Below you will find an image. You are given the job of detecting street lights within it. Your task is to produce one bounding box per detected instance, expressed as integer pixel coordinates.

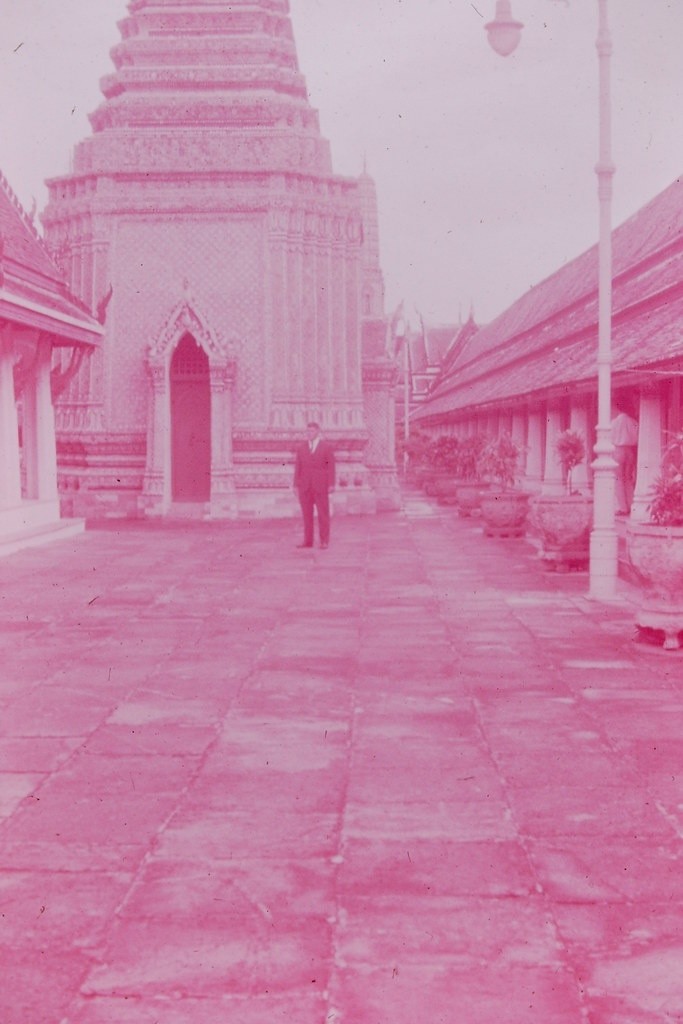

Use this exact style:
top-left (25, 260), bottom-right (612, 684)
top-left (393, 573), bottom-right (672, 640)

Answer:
top-left (485, 0), bottom-right (619, 599)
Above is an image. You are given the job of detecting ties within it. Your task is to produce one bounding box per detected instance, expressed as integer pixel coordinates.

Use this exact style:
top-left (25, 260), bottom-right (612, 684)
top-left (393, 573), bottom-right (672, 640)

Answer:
top-left (310, 440), bottom-right (314, 451)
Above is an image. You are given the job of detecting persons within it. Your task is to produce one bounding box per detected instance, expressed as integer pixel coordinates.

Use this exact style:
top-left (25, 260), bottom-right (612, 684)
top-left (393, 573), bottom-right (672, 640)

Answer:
top-left (294, 423), bottom-right (336, 549)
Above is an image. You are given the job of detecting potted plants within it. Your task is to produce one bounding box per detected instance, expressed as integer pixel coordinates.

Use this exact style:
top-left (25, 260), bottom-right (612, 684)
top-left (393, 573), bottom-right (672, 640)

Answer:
top-left (402, 425), bottom-right (682, 652)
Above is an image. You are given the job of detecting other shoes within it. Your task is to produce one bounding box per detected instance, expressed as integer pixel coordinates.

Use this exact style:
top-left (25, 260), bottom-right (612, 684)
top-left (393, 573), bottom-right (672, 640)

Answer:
top-left (296, 542), bottom-right (313, 548)
top-left (614, 509), bottom-right (631, 515)
top-left (320, 543), bottom-right (329, 549)
